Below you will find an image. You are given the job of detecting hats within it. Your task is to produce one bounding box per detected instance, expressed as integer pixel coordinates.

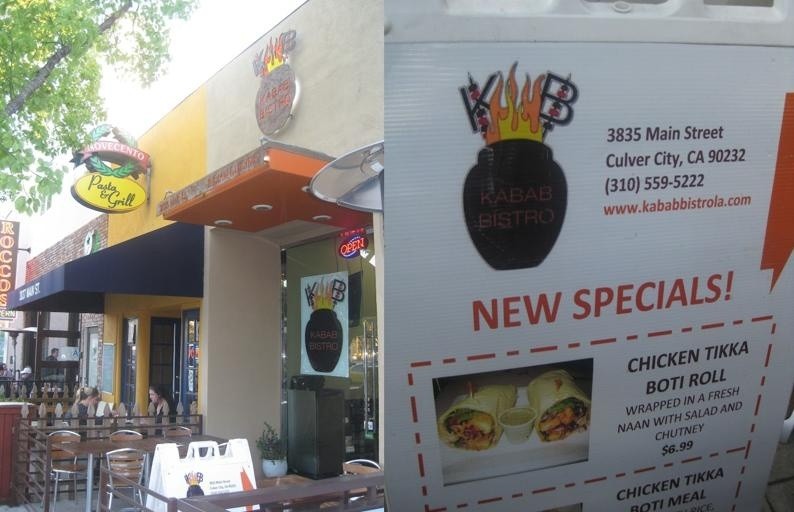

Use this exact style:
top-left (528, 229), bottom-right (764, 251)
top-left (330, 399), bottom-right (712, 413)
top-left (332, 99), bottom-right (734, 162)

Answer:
top-left (18, 367), bottom-right (32, 374)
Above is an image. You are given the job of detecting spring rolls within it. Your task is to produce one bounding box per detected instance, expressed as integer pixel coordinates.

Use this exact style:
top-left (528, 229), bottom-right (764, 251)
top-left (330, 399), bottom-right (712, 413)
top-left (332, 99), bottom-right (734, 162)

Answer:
top-left (528, 369), bottom-right (591, 442)
top-left (437, 383), bottom-right (517, 451)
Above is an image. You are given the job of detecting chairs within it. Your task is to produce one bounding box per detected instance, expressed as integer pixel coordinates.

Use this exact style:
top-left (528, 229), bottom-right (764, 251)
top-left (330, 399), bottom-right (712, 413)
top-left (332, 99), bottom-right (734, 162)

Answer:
top-left (41, 423), bottom-right (380, 511)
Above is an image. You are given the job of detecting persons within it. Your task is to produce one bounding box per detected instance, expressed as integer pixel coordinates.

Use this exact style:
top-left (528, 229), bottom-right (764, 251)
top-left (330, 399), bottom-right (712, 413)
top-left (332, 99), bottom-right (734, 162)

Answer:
top-left (16, 365), bottom-right (35, 395)
top-left (0, 363), bottom-right (12, 378)
top-left (61, 385), bottom-right (117, 487)
top-left (44, 348), bottom-right (59, 361)
top-left (140, 383), bottom-right (175, 435)
